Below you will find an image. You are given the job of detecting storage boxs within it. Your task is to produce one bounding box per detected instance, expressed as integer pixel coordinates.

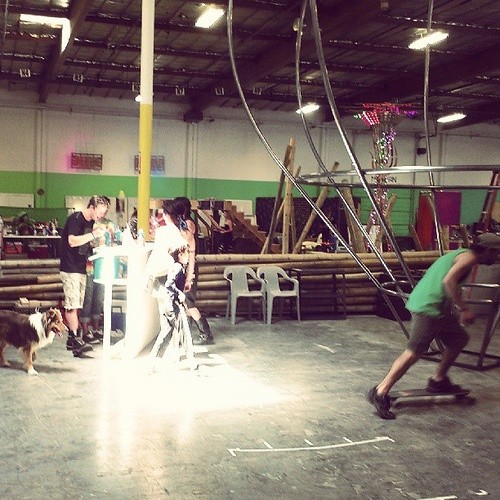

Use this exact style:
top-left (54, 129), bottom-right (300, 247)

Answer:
top-left (376, 274), bottom-right (423, 321)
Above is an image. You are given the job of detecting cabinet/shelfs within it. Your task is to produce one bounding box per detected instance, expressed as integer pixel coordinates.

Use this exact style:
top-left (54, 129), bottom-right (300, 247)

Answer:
top-left (288, 267), bottom-right (346, 320)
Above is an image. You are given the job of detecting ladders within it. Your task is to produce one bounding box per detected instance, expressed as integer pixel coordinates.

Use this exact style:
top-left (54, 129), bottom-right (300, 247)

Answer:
top-left (477, 170), bottom-right (500, 232)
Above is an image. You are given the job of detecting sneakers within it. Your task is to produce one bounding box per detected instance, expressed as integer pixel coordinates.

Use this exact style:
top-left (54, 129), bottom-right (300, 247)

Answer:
top-left (428, 375), bottom-right (460, 393)
top-left (368, 385), bottom-right (395, 419)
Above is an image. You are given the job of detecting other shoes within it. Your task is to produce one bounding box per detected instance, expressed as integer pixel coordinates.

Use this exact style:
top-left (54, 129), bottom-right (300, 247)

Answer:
top-left (83, 332), bottom-right (99, 344)
top-left (72, 337), bottom-right (93, 357)
top-left (67, 336), bottom-right (74, 348)
top-left (94, 329), bottom-right (104, 340)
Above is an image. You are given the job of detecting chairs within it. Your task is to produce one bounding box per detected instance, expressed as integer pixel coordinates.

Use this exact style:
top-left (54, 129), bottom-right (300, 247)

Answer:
top-left (461, 224), bottom-right (473, 248)
top-left (223, 266), bottom-right (266, 326)
top-left (442, 225), bottom-right (464, 250)
top-left (256, 266), bottom-right (300, 325)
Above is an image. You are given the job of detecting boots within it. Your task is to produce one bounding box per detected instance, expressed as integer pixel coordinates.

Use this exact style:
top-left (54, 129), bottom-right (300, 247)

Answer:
top-left (193, 315), bottom-right (213, 344)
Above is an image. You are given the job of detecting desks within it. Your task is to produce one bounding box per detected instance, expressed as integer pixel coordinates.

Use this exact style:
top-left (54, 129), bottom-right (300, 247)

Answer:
top-left (3, 235), bottom-right (62, 259)
top-left (302, 241), bottom-right (321, 250)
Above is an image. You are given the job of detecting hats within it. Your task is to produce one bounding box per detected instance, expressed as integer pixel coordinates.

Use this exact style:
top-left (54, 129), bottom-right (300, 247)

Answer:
top-left (473, 232), bottom-right (500, 249)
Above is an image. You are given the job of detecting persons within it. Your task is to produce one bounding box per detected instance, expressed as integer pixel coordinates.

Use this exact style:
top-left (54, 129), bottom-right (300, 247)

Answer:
top-left (366, 233), bottom-right (500, 420)
top-left (147, 197), bottom-right (200, 373)
top-left (172, 197), bottom-right (214, 344)
top-left (216, 211), bottom-right (234, 254)
top-left (57, 193), bottom-right (112, 357)
top-left (79, 225), bottom-right (107, 344)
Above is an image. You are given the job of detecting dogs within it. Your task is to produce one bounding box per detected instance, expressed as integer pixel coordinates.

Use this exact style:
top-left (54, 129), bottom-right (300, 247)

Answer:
top-left (0, 306), bottom-right (69, 376)
